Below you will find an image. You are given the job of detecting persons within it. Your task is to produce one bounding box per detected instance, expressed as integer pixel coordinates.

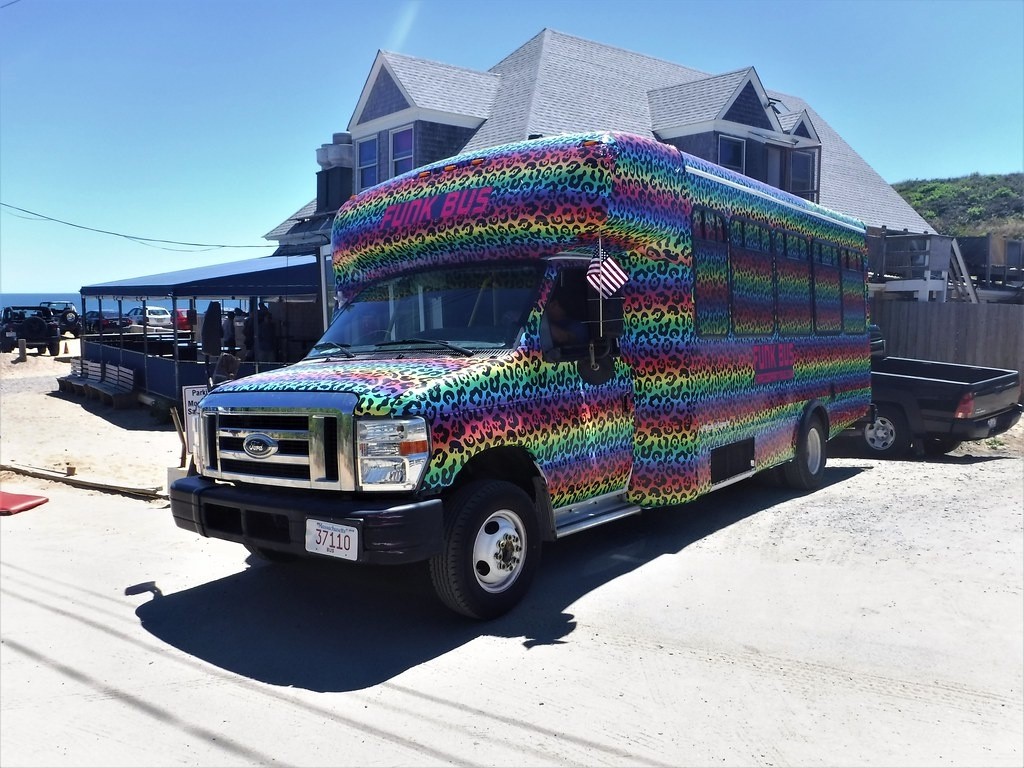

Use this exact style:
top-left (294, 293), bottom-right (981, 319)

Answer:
top-left (499, 270), bottom-right (608, 360)
top-left (243, 312), bottom-right (255, 365)
top-left (222, 311), bottom-right (237, 357)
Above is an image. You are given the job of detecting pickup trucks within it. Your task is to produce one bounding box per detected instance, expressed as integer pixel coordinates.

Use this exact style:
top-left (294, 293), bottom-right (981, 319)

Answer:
top-left (849, 325), bottom-right (1024, 459)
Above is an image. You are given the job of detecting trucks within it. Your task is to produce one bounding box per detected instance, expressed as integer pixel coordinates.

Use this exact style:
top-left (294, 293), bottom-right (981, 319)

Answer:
top-left (164, 125), bottom-right (875, 626)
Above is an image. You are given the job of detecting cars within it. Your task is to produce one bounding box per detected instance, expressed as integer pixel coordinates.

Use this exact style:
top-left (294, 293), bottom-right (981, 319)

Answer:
top-left (168, 309), bottom-right (190, 330)
top-left (79, 309), bottom-right (133, 334)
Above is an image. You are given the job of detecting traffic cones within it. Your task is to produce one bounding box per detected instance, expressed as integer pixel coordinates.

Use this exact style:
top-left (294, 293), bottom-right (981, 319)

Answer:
top-left (63, 341), bottom-right (69, 354)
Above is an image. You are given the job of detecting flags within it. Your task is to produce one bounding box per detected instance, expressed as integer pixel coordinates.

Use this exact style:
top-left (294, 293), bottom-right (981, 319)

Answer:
top-left (585, 240), bottom-right (630, 300)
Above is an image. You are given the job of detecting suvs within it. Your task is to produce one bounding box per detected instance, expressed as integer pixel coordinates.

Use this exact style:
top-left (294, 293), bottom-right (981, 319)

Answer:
top-left (34, 301), bottom-right (83, 338)
top-left (124, 306), bottom-right (173, 329)
top-left (0, 305), bottom-right (61, 356)
